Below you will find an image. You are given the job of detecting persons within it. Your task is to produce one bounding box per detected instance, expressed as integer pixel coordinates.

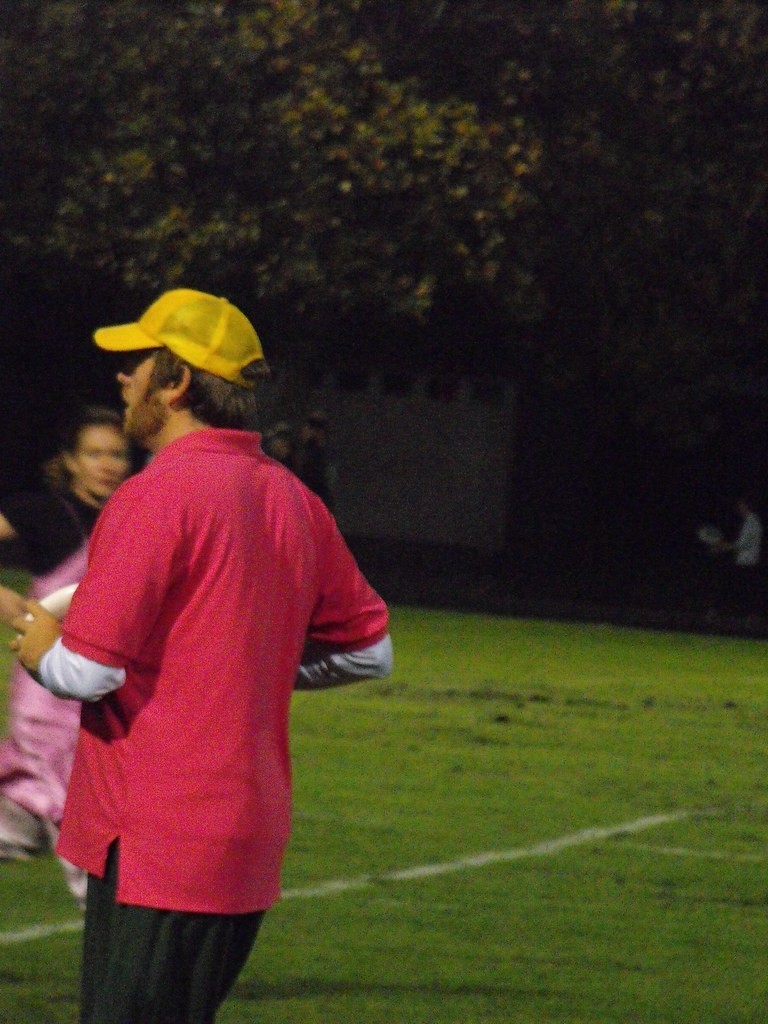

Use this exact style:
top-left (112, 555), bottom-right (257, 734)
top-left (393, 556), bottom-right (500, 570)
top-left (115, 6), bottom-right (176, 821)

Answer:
top-left (0, 289), bottom-right (392, 1024)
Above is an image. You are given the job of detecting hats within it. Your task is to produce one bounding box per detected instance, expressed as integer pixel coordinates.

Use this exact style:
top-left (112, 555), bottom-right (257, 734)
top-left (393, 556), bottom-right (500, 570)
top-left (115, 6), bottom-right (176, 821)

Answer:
top-left (94, 288), bottom-right (264, 391)
